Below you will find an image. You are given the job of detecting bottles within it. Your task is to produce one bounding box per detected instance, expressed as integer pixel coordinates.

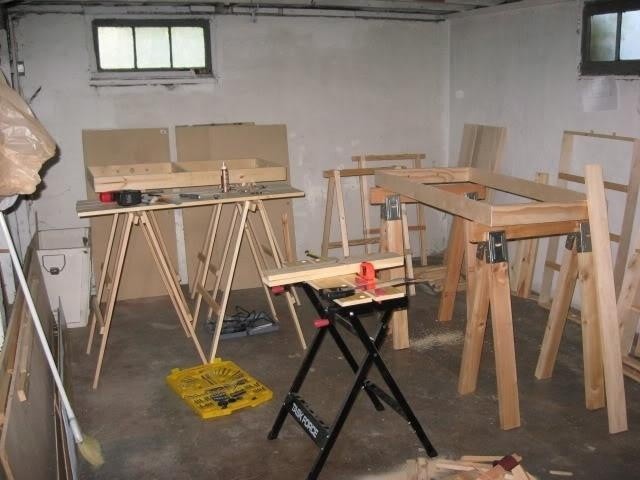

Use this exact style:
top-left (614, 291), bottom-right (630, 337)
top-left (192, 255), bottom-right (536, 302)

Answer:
top-left (220, 162), bottom-right (229, 193)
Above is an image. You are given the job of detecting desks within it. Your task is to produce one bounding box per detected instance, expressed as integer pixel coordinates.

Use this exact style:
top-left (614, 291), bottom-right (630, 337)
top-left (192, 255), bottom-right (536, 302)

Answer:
top-left (76, 181), bottom-right (307, 389)
top-left (269, 256), bottom-right (438, 479)
